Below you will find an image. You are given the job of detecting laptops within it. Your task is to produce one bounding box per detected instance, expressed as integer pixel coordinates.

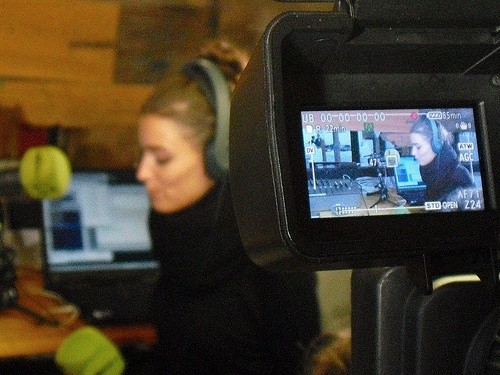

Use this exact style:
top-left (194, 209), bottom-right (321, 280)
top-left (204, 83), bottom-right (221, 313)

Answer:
top-left (393, 155), bottom-right (434, 207)
top-left (39, 171), bottom-right (166, 328)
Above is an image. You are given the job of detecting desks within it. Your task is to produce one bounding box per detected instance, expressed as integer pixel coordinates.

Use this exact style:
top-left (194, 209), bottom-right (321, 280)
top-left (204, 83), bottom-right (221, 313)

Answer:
top-left (0, 268), bottom-right (160, 359)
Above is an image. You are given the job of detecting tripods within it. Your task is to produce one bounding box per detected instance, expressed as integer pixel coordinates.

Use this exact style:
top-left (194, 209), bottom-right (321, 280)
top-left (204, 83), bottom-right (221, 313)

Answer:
top-left (367, 173), bottom-right (401, 209)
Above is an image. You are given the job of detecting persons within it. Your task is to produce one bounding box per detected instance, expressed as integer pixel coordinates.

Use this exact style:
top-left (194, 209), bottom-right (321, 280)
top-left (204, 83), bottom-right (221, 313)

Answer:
top-left (409, 114), bottom-right (476, 206)
top-left (119, 40), bottom-right (322, 375)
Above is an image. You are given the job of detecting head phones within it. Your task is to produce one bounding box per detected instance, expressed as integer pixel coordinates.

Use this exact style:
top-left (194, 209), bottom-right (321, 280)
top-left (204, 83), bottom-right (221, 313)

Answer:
top-left (423, 116), bottom-right (445, 152)
top-left (187, 59), bottom-right (234, 179)
